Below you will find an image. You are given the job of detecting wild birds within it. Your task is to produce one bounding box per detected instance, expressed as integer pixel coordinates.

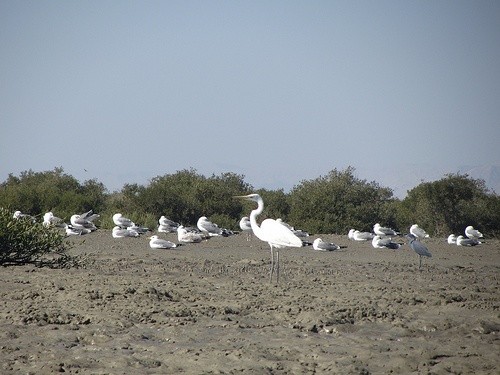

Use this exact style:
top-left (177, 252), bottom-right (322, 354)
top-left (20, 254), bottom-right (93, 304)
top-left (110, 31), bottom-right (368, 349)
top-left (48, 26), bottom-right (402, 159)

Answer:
top-left (233, 194), bottom-right (303, 286)
top-left (12, 209), bottom-right (483, 269)
